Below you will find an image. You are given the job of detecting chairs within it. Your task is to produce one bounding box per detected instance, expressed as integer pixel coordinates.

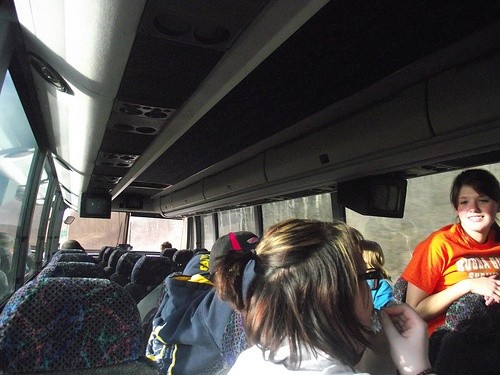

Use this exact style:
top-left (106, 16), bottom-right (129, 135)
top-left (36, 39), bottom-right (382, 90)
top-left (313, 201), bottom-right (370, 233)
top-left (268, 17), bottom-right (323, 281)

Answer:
top-left (0, 247), bottom-right (500, 375)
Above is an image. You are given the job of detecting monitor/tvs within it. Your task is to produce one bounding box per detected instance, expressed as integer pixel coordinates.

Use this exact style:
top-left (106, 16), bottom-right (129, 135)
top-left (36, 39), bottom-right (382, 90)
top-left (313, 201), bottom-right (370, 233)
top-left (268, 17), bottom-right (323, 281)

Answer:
top-left (80, 191), bottom-right (111, 219)
top-left (336, 173), bottom-right (407, 218)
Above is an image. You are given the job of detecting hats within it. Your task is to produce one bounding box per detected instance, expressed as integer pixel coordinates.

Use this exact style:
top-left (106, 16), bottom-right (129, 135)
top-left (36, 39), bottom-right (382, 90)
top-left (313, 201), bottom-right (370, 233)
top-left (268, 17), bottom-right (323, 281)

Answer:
top-left (210, 231), bottom-right (261, 274)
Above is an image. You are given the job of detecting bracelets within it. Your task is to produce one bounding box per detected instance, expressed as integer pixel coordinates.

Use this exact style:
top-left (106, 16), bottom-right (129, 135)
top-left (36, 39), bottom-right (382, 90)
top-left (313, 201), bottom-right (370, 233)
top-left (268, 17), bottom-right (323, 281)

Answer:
top-left (418, 368), bottom-right (437, 375)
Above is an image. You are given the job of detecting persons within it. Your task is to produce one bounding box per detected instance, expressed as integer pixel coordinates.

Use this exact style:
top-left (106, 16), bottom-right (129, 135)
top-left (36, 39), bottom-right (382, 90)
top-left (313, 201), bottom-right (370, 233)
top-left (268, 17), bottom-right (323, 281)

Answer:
top-left (161, 241), bottom-right (172, 252)
top-left (401, 168), bottom-right (500, 336)
top-left (0, 232), bottom-right (29, 275)
top-left (146, 231), bottom-right (261, 375)
top-left (360, 240), bottom-right (395, 314)
top-left (216, 218), bottom-right (437, 375)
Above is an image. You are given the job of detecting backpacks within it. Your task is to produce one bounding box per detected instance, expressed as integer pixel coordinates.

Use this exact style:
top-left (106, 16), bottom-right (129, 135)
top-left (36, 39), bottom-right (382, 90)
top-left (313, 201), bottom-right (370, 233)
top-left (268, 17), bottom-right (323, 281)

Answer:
top-left (152, 255), bottom-right (214, 328)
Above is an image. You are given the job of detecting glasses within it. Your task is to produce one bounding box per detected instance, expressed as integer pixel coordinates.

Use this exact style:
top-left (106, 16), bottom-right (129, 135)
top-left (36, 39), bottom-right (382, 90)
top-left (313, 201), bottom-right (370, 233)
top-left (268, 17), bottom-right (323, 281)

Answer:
top-left (357, 270), bottom-right (380, 291)
